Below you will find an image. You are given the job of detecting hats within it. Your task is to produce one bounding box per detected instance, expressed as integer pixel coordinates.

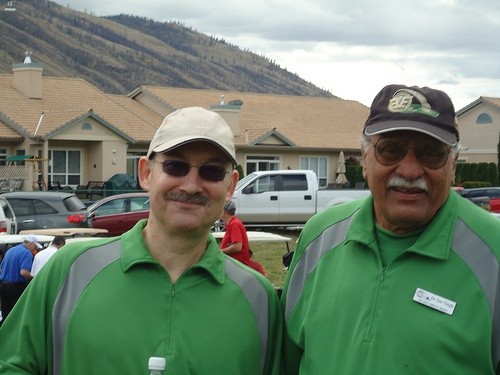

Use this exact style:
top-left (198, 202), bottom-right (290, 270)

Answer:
top-left (25, 235), bottom-right (43, 248)
top-left (147, 106), bottom-right (238, 168)
top-left (362, 84), bottom-right (460, 147)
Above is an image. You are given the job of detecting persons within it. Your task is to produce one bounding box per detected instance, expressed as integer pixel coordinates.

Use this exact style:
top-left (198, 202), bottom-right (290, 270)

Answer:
top-left (280, 84), bottom-right (500, 375)
top-left (0, 234), bottom-right (65, 326)
top-left (0, 106), bottom-right (279, 375)
top-left (220, 201), bottom-right (265, 275)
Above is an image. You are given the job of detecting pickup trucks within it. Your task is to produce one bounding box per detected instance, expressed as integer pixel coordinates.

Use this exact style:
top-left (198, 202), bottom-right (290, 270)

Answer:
top-left (222, 170), bottom-right (372, 230)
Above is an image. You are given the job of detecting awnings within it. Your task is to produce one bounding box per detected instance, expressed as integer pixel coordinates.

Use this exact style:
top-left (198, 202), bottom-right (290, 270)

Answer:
top-left (4, 155), bottom-right (34, 160)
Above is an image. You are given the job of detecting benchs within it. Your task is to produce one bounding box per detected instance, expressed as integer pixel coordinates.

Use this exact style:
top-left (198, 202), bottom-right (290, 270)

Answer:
top-left (38, 180), bottom-right (73, 193)
top-left (75, 181), bottom-right (106, 201)
top-left (0, 179), bottom-right (24, 194)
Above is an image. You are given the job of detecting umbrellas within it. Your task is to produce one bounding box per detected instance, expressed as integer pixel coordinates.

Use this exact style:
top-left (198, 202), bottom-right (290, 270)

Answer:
top-left (335, 150), bottom-right (349, 187)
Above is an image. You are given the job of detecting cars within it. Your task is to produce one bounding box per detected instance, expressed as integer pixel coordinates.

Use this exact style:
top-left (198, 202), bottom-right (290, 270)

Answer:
top-left (451, 186), bottom-right (500, 212)
top-left (87, 192), bottom-right (153, 237)
top-left (1, 192), bottom-right (89, 234)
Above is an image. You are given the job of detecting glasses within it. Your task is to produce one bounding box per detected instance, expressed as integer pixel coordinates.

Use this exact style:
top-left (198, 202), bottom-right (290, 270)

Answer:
top-left (153, 159), bottom-right (234, 182)
top-left (368, 137), bottom-right (452, 170)
